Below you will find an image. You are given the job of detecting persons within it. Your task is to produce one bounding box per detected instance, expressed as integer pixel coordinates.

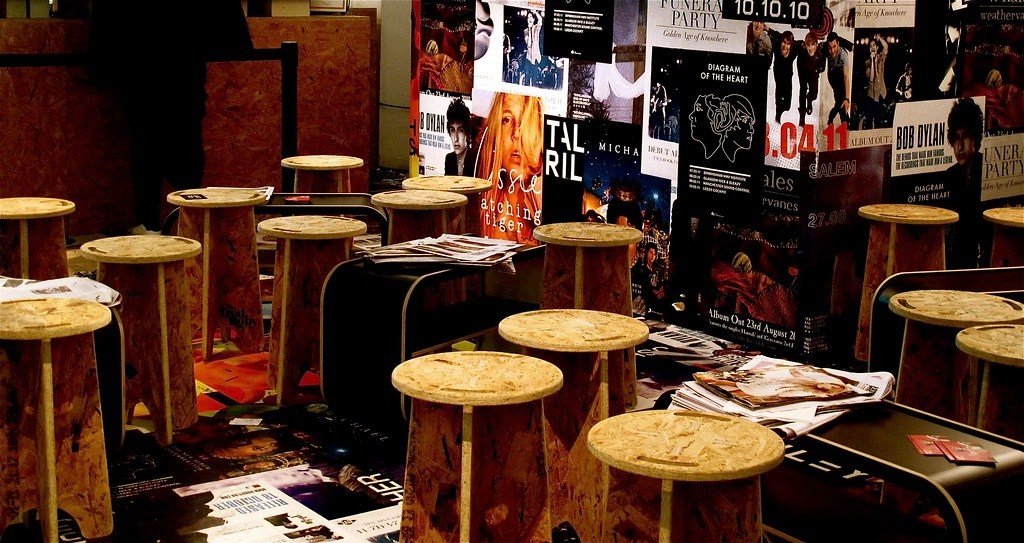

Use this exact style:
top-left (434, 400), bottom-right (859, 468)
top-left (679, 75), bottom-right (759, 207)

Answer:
top-left (944, 97), bottom-right (983, 201)
top-left (474, 92), bottom-right (544, 245)
top-left (524, 9), bottom-right (542, 86)
top-left (49, 0), bottom-right (257, 243)
top-left (746, 21), bottom-right (912, 130)
top-left (716, 365), bottom-right (849, 397)
top-left (634, 242), bottom-right (663, 321)
top-left (444, 97), bottom-right (483, 175)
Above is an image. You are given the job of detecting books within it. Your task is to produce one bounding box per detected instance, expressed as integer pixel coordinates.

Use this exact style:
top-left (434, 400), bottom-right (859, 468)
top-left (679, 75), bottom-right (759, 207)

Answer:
top-left (355, 233), bottom-right (526, 267)
top-left (693, 354), bottom-right (873, 410)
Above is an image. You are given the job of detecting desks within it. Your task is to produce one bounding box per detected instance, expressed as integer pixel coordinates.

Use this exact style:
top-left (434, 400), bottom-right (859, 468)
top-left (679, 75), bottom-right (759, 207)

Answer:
top-left (868, 267), bottom-right (1024, 401)
top-left (318, 234), bottom-right (546, 424)
top-left (0, 275), bottom-right (126, 450)
top-left (652, 388), bottom-right (1024, 543)
top-left (161, 193), bottom-right (389, 247)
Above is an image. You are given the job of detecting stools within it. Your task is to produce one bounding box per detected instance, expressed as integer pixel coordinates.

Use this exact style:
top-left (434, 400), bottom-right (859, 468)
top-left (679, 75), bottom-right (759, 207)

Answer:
top-left (854, 204), bottom-right (959, 362)
top-left (0, 197), bottom-right (75, 281)
top-left (889, 290), bottom-right (1024, 427)
top-left (402, 176), bottom-right (492, 235)
top-left (0, 298), bottom-right (113, 543)
top-left (956, 324), bottom-right (1024, 443)
top-left (983, 207), bottom-right (1024, 268)
top-left (586, 410), bottom-right (785, 543)
top-left (257, 215), bottom-right (367, 407)
top-left (392, 350), bottom-right (563, 543)
top-left (281, 155), bottom-right (364, 251)
top-left (371, 190), bottom-right (468, 246)
top-left (533, 222), bottom-right (644, 408)
top-left (167, 188), bottom-right (266, 360)
top-left (80, 235), bottom-right (203, 446)
top-left (499, 309), bottom-right (649, 543)
